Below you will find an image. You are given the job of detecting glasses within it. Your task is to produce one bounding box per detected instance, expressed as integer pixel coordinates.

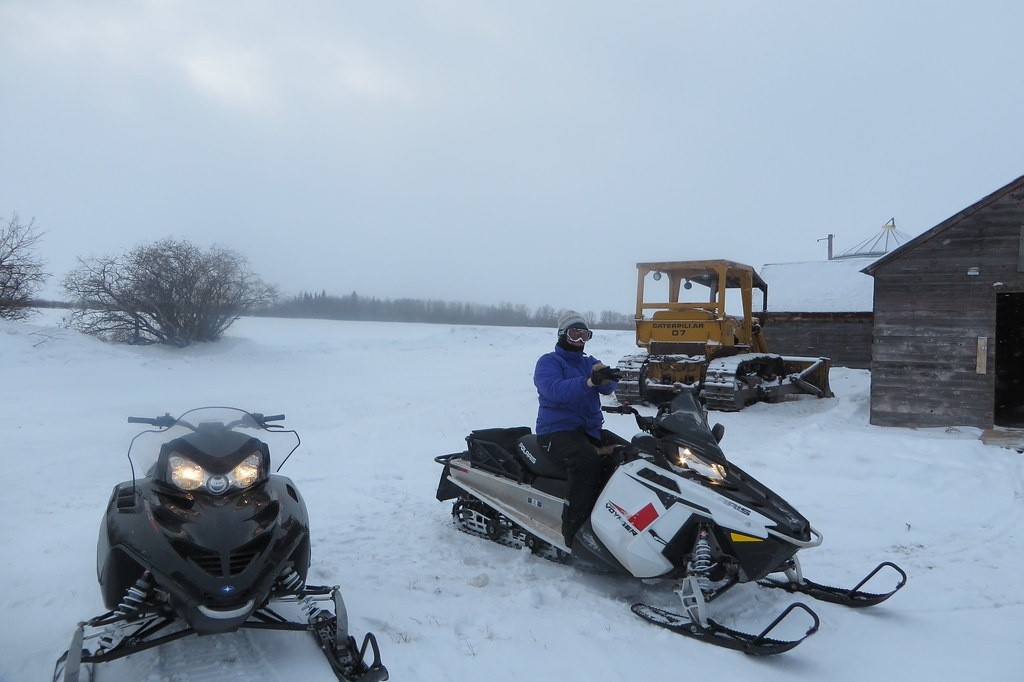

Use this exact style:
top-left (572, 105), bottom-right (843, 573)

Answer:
top-left (558, 327), bottom-right (593, 343)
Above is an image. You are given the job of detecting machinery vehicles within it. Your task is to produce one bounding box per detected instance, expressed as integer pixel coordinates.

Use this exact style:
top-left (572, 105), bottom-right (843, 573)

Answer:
top-left (612, 259), bottom-right (834, 413)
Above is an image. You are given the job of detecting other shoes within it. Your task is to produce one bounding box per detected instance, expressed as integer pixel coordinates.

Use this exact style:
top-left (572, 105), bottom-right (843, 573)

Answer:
top-left (565, 519), bottom-right (579, 533)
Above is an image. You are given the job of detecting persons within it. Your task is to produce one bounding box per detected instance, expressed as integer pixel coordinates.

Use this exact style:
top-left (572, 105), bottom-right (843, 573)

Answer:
top-left (533, 310), bottom-right (630, 548)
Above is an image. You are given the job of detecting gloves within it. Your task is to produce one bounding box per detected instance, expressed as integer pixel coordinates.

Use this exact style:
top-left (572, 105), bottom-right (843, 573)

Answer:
top-left (591, 366), bottom-right (622, 385)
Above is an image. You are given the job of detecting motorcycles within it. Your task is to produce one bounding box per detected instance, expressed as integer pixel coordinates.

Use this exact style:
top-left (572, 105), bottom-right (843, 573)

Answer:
top-left (434, 390), bottom-right (907, 656)
top-left (53, 408), bottom-right (389, 681)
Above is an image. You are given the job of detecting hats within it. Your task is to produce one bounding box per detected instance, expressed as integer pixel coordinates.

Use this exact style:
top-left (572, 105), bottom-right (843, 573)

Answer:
top-left (558, 310), bottom-right (588, 340)
